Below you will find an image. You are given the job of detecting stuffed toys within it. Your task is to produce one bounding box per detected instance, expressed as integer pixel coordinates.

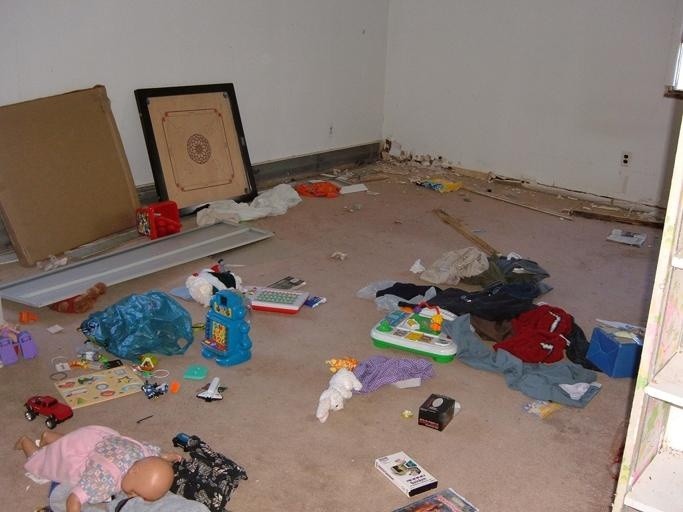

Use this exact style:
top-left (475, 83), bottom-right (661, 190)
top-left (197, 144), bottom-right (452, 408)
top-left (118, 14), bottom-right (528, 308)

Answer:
top-left (185, 259), bottom-right (243, 308)
top-left (46, 280), bottom-right (108, 314)
top-left (314, 366), bottom-right (362, 425)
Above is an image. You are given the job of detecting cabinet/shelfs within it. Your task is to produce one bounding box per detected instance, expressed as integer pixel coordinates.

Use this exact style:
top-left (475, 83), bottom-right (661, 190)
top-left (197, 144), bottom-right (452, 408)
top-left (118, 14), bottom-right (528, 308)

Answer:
top-left (622, 251), bottom-right (683, 512)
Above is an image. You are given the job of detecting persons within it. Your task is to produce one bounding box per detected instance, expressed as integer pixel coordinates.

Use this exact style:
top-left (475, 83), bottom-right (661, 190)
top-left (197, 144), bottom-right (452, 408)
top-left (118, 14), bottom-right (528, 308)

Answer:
top-left (12, 423), bottom-right (183, 511)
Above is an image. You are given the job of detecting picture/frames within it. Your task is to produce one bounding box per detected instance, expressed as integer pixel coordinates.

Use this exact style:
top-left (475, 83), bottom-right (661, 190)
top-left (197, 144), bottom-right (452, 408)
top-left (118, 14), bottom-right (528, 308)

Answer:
top-left (132, 82), bottom-right (257, 220)
